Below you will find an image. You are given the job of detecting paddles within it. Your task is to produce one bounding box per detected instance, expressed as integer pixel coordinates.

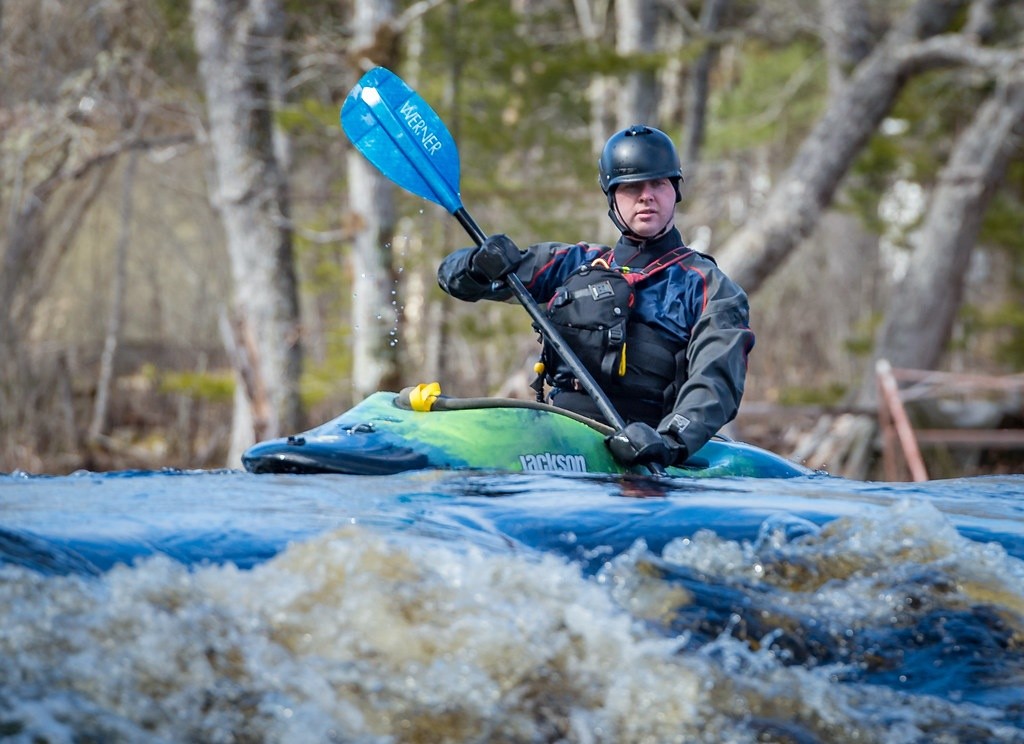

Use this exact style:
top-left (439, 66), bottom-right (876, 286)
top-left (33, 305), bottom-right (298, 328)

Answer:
top-left (335, 62), bottom-right (674, 482)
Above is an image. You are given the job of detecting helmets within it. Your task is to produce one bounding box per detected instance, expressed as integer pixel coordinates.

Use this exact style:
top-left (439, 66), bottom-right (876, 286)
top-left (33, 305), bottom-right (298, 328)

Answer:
top-left (598, 124), bottom-right (683, 204)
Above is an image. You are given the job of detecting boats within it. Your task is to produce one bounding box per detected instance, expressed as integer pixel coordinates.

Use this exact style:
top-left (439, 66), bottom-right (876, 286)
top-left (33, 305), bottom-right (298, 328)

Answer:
top-left (240, 388), bottom-right (816, 480)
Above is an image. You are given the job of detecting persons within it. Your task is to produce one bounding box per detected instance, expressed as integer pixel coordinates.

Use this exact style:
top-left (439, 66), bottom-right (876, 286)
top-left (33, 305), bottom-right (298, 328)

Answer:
top-left (439, 125), bottom-right (756, 468)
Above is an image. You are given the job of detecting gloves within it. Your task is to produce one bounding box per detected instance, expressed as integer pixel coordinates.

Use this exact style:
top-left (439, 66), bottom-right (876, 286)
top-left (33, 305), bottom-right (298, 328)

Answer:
top-left (466, 233), bottom-right (522, 292)
top-left (604, 422), bottom-right (687, 469)
